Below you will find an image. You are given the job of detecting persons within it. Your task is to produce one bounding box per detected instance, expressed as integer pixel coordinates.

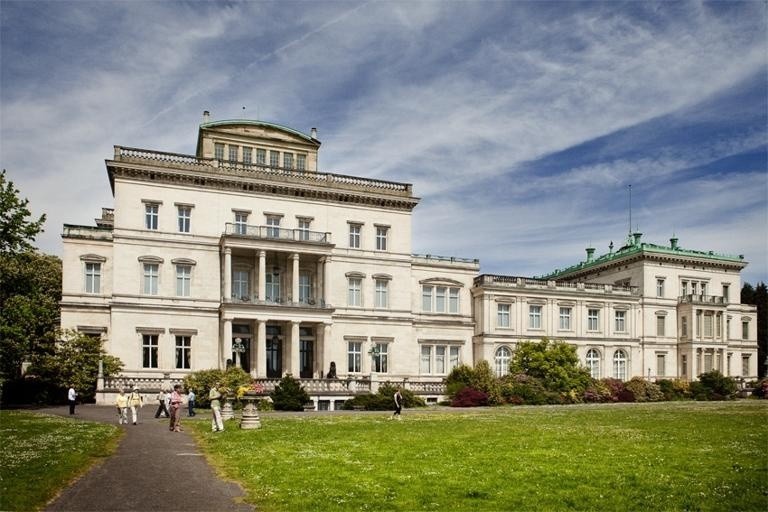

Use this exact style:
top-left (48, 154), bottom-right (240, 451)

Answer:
top-left (154, 388), bottom-right (171, 419)
top-left (392, 387), bottom-right (403, 416)
top-left (160, 389), bottom-right (172, 417)
top-left (127, 386), bottom-right (144, 426)
top-left (68, 382), bottom-right (77, 415)
top-left (168, 384), bottom-right (183, 431)
top-left (207, 381), bottom-right (227, 432)
top-left (186, 387), bottom-right (197, 416)
top-left (115, 387), bottom-right (129, 423)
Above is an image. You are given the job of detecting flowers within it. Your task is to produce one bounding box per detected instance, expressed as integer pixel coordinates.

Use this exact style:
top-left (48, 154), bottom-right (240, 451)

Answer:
top-left (218, 386), bottom-right (234, 394)
top-left (238, 383), bottom-right (264, 400)
